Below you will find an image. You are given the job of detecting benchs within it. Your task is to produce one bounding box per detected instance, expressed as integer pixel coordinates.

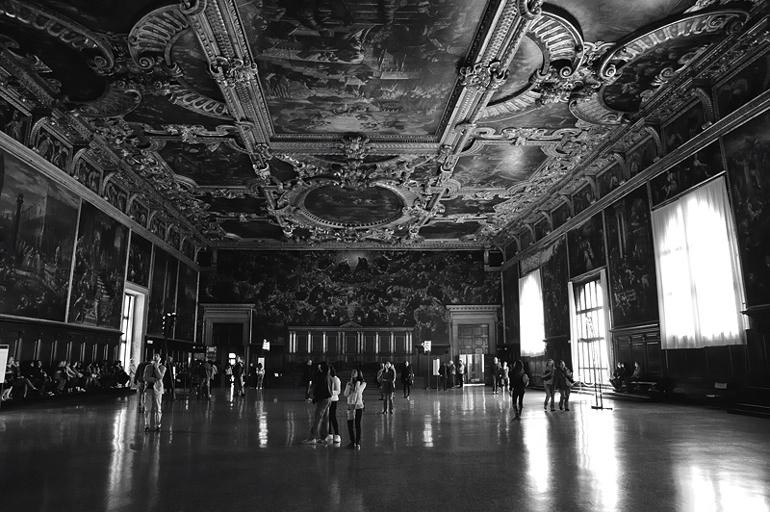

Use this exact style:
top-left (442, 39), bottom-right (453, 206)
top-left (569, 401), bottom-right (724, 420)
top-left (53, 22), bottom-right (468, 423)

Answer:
top-left (613, 378), bottom-right (657, 393)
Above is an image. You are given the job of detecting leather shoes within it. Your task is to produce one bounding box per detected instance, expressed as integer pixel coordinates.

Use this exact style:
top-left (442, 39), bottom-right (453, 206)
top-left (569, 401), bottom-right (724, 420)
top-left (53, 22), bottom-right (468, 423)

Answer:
top-left (144, 425), bottom-right (148, 431)
top-left (155, 425), bottom-right (160, 430)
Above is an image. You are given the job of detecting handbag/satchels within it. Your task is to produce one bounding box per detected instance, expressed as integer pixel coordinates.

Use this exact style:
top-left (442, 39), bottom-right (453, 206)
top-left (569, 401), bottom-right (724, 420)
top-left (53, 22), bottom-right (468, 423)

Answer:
top-left (523, 373), bottom-right (529, 385)
top-left (565, 376), bottom-right (573, 387)
top-left (346, 409), bottom-right (356, 421)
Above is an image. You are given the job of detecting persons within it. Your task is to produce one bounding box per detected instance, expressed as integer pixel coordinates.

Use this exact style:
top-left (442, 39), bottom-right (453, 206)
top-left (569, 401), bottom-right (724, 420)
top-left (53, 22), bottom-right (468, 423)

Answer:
top-left (344, 368), bottom-right (368, 451)
top-left (630, 360), bottom-right (641, 390)
top-left (0, 350), bottom-right (263, 436)
top-left (617, 363), bottom-right (627, 387)
top-left (324, 364), bottom-right (343, 444)
top-left (305, 359), bottom-right (574, 420)
top-left (305, 362), bottom-right (331, 445)
top-left (610, 361), bottom-right (620, 389)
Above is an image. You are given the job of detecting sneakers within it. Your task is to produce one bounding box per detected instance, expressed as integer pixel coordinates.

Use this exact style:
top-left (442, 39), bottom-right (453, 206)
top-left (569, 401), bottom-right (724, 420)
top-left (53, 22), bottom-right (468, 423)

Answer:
top-left (301, 431), bottom-right (361, 450)
